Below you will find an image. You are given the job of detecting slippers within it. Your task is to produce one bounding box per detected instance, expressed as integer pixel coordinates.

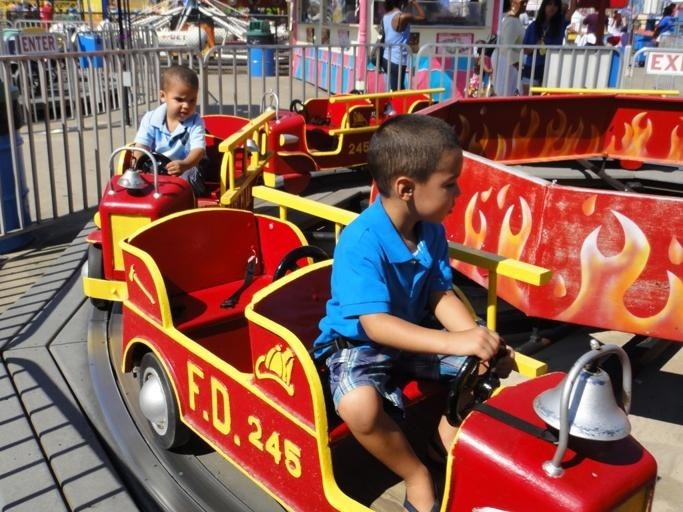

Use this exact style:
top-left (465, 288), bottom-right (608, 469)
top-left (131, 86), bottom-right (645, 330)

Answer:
top-left (402, 472), bottom-right (440, 512)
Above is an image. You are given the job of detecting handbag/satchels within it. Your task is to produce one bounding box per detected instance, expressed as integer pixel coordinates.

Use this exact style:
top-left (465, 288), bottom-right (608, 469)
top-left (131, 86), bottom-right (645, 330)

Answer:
top-left (371, 35), bottom-right (384, 67)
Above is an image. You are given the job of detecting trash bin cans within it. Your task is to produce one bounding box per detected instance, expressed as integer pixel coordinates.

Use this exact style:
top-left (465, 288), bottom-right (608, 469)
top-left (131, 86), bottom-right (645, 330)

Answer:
top-left (77, 33), bottom-right (104, 70)
top-left (243, 20), bottom-right (275, 77)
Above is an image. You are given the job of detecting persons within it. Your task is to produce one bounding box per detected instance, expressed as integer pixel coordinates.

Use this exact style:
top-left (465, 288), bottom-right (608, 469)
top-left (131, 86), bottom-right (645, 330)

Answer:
top-left (380, 0), bottom-right (427, 92)
top-left (132, 65), bottom-right (209, 194)
top-left (8, 0), bottom-right (83, 30)
top-left (312, 114), bottom-right (515, 512)
top-left (485, 0), bottom-right (683, 97)
